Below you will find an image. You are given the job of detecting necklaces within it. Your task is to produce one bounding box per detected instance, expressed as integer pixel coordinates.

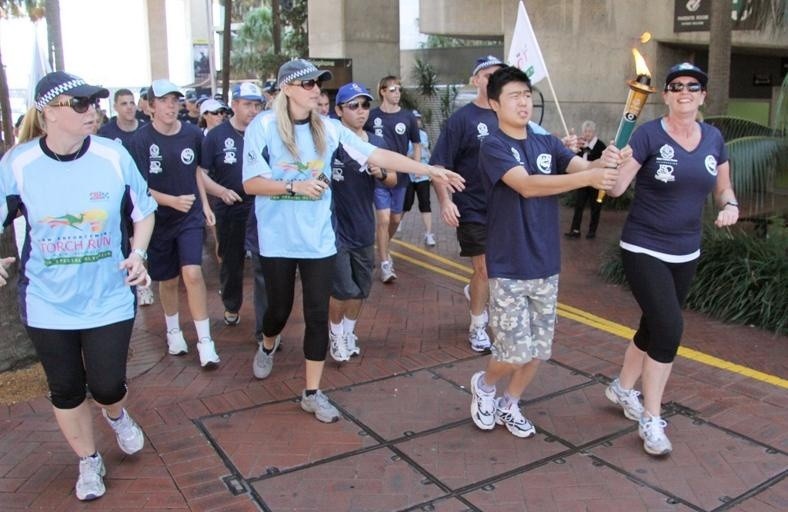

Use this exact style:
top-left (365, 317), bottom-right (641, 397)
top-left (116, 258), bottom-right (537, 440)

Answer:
top-left (46, 138), bottom-right (80, 174)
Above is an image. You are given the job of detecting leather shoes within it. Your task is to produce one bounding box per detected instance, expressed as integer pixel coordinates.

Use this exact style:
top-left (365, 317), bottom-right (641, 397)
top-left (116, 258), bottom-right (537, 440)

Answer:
top-left (586, 233), bottom-right (596, 239)
top-left (564, 230), bottom-right (581, 239)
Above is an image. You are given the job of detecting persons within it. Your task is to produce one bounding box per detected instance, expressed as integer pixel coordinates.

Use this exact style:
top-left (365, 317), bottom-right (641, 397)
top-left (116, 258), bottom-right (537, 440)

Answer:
top-left (199, 98), bottom-right (225, 265)
top-left (137, 87), bottom-right (153, 123)
top-left (329, 83), bottom-right (398, 363)
top-left (1, 72), bottom-right (158, 502)
top-left (363, 77), bottom-right (420, 283)
top-left (318, 89), bottom-right (339, 119)
top-left (564, 120), bottom-right (607, 241)
top-left (601, 62), bottom-right (738, 456)
top-left (200, 81), bottom-right (284, 351)
top-left (394, 109), bottom-right (437, 247)
top-left (428, 56), bottom-right (577, 353)
top-left (470, 66), bottom-right (634, 440)
top-left (96, 87), bottom-right (154, 307)
top-left (180, 90), bottom-right (199, 124)
top-left (130, 79), bottom-right (221, 371)
top-left (242, 57), bottom-right (465, 423)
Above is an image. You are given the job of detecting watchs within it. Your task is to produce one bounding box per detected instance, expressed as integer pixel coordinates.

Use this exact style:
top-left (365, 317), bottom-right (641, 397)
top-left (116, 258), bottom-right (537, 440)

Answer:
top-left (376, 167), bottom-right (388, 182)
top-left (132, 247), bottom-right (148, 260)
top-left (720, 201), bottom-right (738, 211)
top-left (285, 180), bottom-right (295, 197)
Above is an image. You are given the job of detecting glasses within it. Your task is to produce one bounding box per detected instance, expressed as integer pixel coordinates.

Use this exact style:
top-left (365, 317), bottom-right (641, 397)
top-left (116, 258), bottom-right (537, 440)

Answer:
top-left (664, 81), bottom-right (707, 92)
top-left (140, 96), bottom-right (149, 101)
top-left (207, 109), bottom-right (225, 116)
top-left (47, 96), bottom-right (98, 112)
top-left (380, 86), bottom-right (404, 94)
top-left (339, 101), bottom-right (373, 112)
top-left (287, 79), bottom-right (325, 93)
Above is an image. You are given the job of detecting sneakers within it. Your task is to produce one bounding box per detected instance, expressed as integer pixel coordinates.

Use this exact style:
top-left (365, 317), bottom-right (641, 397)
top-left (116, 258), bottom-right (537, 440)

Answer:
top-left (424, 233), bottom-right (436, 247)
top-left (468, 370), bottom-right (497, 432)
top-left (327, 330), bottom-right (352, 363)
top-left (463, 282), bottom-right (475, 312)
top-left (101, 404), bottom-right (146, 457)
top-left (467, 324), bottom-right (493, 354)
top-left (253, 333), bottom-right (282, 380)
top-left (344, 331), bottom-right (361, 358)
top-left (222, 310), bottom-right (241, 326)
top-left (638, 414), bottom-right (675, 458)
top-left (75, 449), bottom-right (107, 501)
top-left (165, 328), bottom-right (189, 357)
top-left (138, 288), bottom-right (154, 307)
top-left (380, 261), bottom-right (398, 284)
top-left (394, 219), bottom-right (405, 240)
top-left (605, 377), bottom-right (644, 423)
top-left (196, 340), bottom-right (222, 370)
top-left (301, 388), bottom-right (341, 425)
top-left (494, 396), bottom-right (538, 440)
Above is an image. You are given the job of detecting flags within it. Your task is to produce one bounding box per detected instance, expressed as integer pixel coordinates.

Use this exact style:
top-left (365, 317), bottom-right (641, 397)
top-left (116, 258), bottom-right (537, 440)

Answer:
top-left (501, 2), bottom-right (548, 87)
top-left (28, 35), bottom-right (52, 109)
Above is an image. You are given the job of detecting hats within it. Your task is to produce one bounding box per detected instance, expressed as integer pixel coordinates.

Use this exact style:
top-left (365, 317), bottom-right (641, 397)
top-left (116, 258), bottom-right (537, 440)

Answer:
top-left (199, 100), bottom-right (229, 117)
top-left (141, 87), bottom-right (153, 97)
top-left (472, 55), bottom-right (509, 75)
top-left (262, 81), bottom-right (279, 94)
top-left (145, 79), bottom-right (185, 107)
top-left (230, 81), bottom-right (265, 104)
top-left (663, 61), bottom-right (712, 87)
top-left (276, 57), bottom-right (335, 91)
top-left (335, 83), bottom-right (375, 107)
top-left (33, 71), bottom-right (109, 113)
